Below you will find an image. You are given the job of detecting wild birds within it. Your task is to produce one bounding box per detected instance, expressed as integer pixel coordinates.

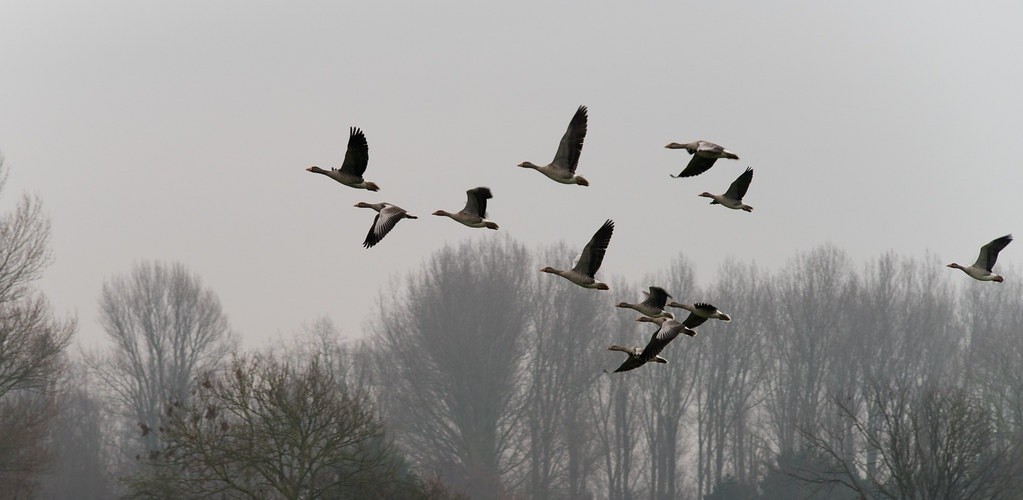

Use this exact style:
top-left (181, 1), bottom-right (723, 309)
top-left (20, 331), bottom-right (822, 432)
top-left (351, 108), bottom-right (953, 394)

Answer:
top-left (540, 221), bottom-right (616, 292)
top-left (945, 233), bottom-right (1014, 284)
top-left (352, 200), bottom-right (419, 250)
top-left (605, 285), bottom-right (732, 375)
top-left (516, 103), bottom-right (590, 187)
top-left (305, 125), bottom-right (381, 193)
top-left (431, 185), bottom-right (499, 231)
top-left (697, 166), bottom-right (754, 215)
top-left (663, 139), bottom-right (740, 178)
